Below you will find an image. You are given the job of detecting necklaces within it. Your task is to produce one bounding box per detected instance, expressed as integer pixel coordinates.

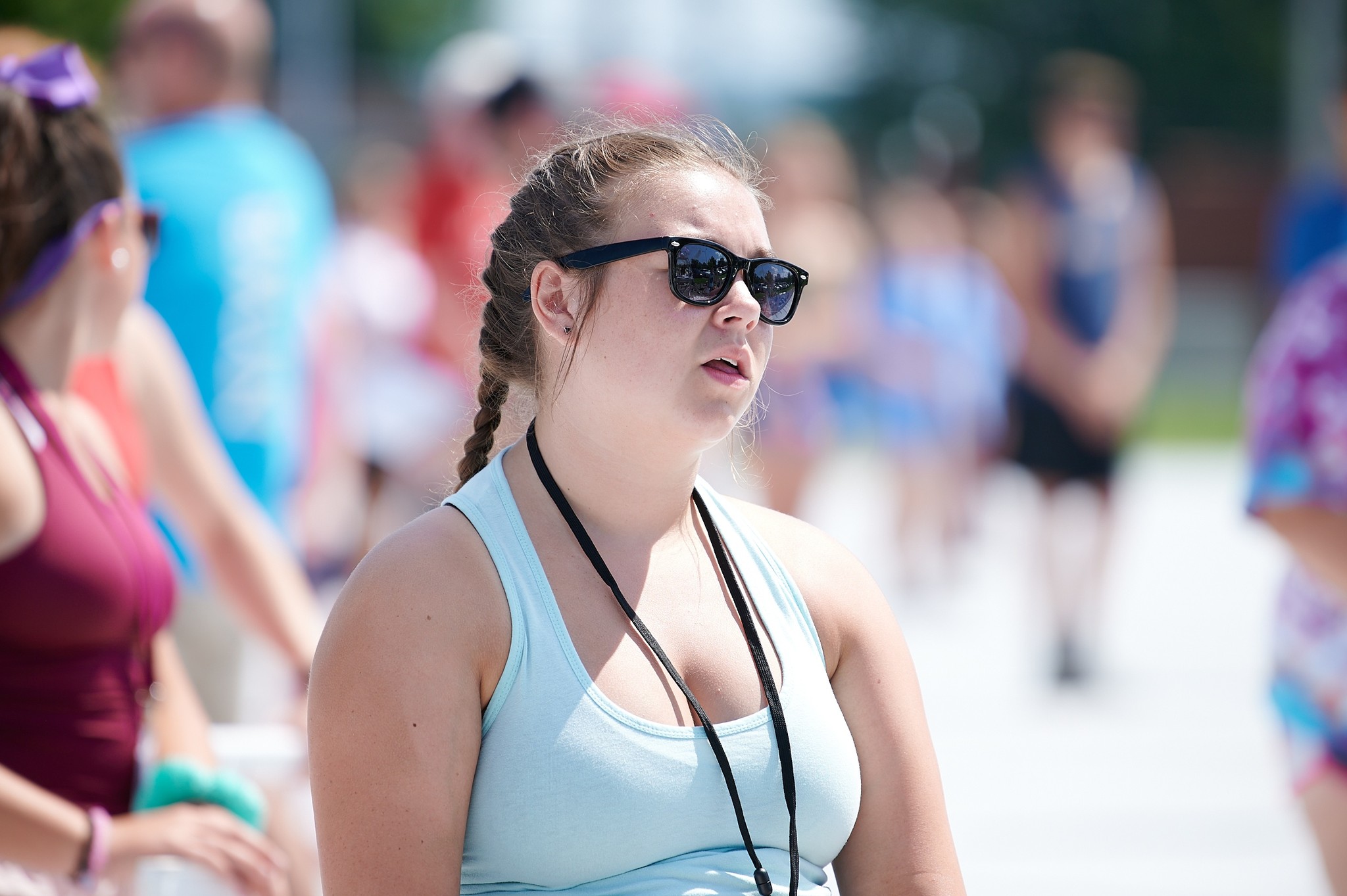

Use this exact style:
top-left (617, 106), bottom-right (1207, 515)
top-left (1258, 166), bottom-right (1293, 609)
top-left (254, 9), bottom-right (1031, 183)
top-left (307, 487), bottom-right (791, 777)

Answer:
top-left (527, 421), bottom-right (798, 896)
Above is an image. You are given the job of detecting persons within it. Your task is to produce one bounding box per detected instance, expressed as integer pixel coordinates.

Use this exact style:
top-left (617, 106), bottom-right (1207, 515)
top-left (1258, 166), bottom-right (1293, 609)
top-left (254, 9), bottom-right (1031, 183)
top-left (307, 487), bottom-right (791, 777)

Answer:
top-left (0, 3), bottom-right (1178, 896)
top-left (1240, 61), bottom-right (1346, 891)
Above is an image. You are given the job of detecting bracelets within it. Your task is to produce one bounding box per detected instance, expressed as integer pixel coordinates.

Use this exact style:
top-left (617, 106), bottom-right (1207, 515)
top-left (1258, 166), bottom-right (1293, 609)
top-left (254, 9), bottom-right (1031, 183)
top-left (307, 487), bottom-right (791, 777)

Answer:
top-left (68, 804), bottom-right (111, 887)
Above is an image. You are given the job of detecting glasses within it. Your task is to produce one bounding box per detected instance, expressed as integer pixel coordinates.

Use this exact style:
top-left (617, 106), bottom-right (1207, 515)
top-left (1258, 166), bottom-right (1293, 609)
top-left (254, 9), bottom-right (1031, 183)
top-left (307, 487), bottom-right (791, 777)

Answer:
top-left (556, 234), bottom-right (810, 325)
top-left (137, 212), bottom-right (158, 244)
